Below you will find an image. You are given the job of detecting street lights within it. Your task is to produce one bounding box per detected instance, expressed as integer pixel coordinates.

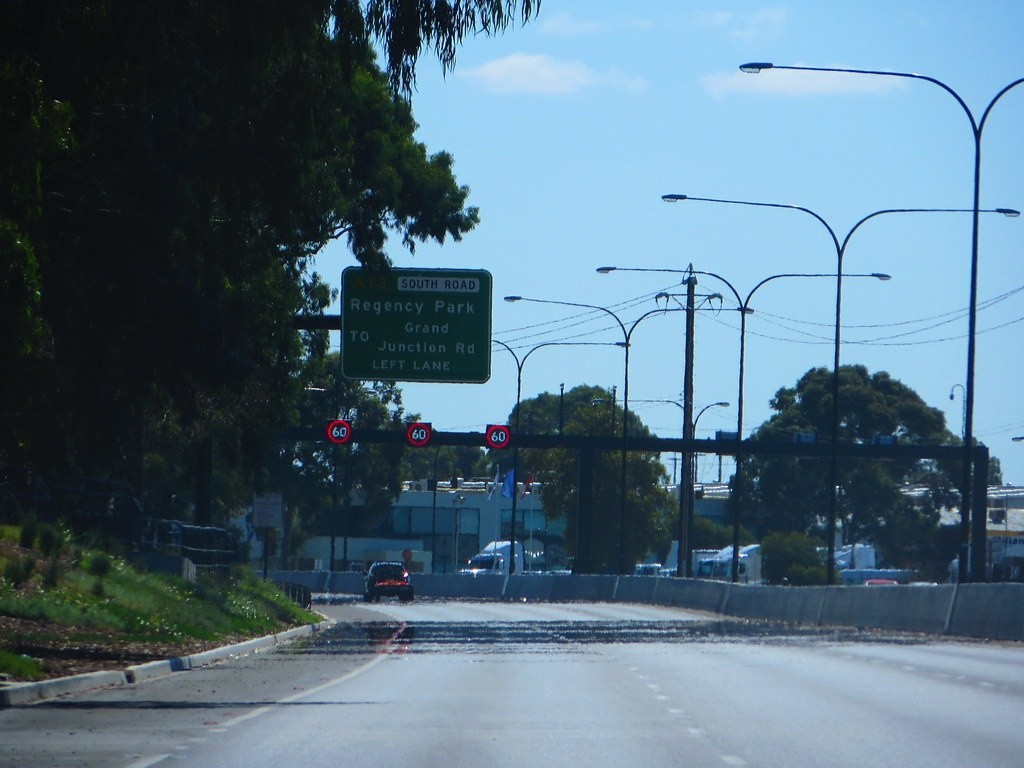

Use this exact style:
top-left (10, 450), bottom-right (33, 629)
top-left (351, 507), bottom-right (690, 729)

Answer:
top-left (596, 267), bottom-right (892, 582)
top-left (590, 399), bottom-right (730, 575)
top-left (491, 339), bottom-right (633, 574)
top-left (739, 62), bottom-right (1023, 584)
top-left (503, 296), bottom-right (755, 574)
top-left (661, 194), bottom-right (1021, 585)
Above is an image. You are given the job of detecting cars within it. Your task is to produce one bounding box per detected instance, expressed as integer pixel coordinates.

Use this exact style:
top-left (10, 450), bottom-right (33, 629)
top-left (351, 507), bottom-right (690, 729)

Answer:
top-left (363, 561), bottom-right (415, 601)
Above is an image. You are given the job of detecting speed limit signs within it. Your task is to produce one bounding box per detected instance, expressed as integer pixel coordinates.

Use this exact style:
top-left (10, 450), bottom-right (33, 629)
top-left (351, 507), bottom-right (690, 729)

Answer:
top-left (406, 422), bottom-right (432, 447)
top-left (486, 424), bottom-right (512, 449)
top-left (326, 419), bottom-right (352, 446)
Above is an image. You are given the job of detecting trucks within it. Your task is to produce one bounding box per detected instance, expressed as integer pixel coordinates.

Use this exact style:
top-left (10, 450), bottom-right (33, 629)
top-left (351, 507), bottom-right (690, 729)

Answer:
top-left (469, 541), bottom-right (524, 573)
top-left (635, 544), bottom-right (941, 586)
